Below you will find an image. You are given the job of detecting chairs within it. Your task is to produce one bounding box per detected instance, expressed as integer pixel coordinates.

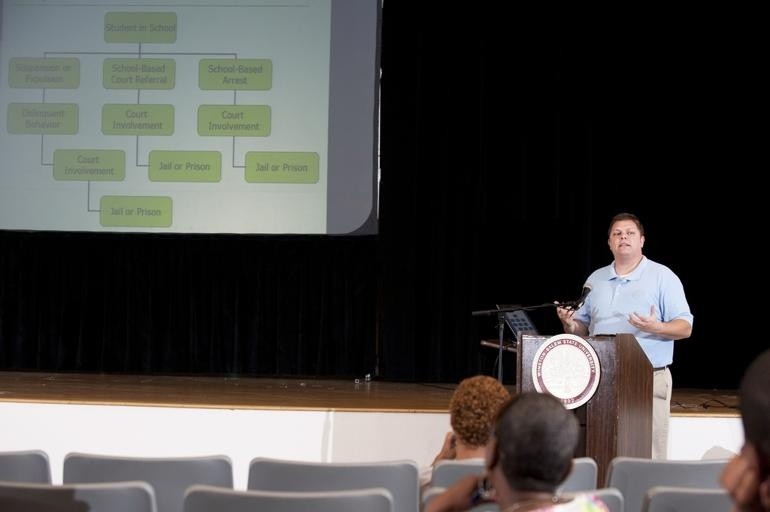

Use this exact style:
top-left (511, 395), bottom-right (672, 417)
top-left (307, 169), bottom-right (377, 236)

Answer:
top-left (0, 479), bottom-right (158, 512)
top-left (0, 448), bottom-right (52, 484)
top-left (62, 451), bottom-right (234, 512)
top-left (422, 486), bottom-right (624, 512)
top-left (640, 485), bottom-right (738, 512)
top-left (180, 483), bottom-right (397, 512)
top-left (604, 456), bottom-right (731, 512)
top-left (248, 456), bottom-right (421, 512)
top-left (430, 456), bottom-right (599, 495)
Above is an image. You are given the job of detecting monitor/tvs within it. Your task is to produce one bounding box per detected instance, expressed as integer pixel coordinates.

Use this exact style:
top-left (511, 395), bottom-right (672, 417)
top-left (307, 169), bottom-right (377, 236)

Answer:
top-left (495, 303), bottom-right (540, 339)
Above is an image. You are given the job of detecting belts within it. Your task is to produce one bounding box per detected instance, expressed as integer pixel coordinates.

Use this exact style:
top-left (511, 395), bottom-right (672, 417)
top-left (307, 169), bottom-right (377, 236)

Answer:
top-left (652, 366), bottom-right (669, 372)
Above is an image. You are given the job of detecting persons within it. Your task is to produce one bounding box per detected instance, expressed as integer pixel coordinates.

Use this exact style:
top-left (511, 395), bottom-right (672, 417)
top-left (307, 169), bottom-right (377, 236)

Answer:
top-left (426, 391), bottom-right (608, 512)
top-left (553, 214), bottom-right (694, 458)
top-left (721, 349), bottom-right (770, 512)
top-left (419, 375), bottom-right (509, 511)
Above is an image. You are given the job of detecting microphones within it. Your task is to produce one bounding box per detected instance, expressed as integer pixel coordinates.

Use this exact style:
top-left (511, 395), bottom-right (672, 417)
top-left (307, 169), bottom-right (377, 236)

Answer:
top-left (572, 282), bottom-right (592, 310)
